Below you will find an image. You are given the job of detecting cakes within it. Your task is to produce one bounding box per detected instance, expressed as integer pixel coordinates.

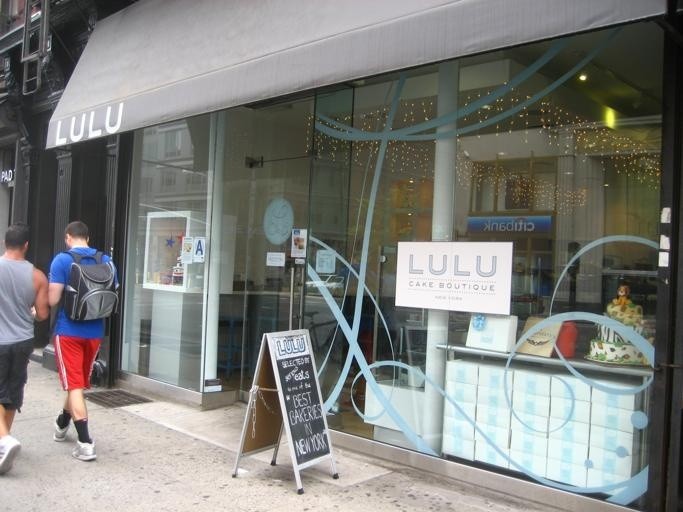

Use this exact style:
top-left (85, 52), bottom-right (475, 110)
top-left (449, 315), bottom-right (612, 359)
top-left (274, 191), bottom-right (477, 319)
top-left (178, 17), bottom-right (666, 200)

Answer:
top-left (590, 286), bottom-right (655, 365)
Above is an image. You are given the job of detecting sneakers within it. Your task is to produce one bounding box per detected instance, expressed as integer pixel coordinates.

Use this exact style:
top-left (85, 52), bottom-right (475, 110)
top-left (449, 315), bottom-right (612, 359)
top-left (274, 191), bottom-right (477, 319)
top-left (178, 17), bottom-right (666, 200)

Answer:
top-left (53, 409), bottom-right (70, 442)
top-left (0, 435), bottom-right (22, 474)
top-left (72, 435), bottom-right (96, 461)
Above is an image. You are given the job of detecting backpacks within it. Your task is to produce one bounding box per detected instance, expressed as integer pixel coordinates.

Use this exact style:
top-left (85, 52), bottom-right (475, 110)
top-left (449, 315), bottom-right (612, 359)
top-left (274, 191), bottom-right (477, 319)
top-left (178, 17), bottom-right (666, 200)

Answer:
top-left (64, 249), bottom-right (120, 320)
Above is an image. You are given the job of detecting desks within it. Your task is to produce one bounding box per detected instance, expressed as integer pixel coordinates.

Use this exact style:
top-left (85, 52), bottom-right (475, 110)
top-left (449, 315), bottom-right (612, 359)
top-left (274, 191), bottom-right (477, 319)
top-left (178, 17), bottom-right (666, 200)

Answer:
top-left (437, 342), bottom-right (653, 509)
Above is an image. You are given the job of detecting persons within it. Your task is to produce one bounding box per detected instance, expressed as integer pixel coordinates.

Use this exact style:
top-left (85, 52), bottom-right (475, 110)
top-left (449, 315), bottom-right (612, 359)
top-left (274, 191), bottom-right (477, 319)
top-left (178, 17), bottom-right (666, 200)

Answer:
top-left (47, 220), bottom-right (117, 463)
top-left (0, 222), bottom-right (49, 475)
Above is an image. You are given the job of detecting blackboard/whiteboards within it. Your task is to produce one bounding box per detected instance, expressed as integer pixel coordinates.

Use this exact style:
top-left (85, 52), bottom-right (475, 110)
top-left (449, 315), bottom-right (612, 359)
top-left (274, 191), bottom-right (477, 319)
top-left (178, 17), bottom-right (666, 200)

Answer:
top-left (266, 328), bottom-right (333, 471)
